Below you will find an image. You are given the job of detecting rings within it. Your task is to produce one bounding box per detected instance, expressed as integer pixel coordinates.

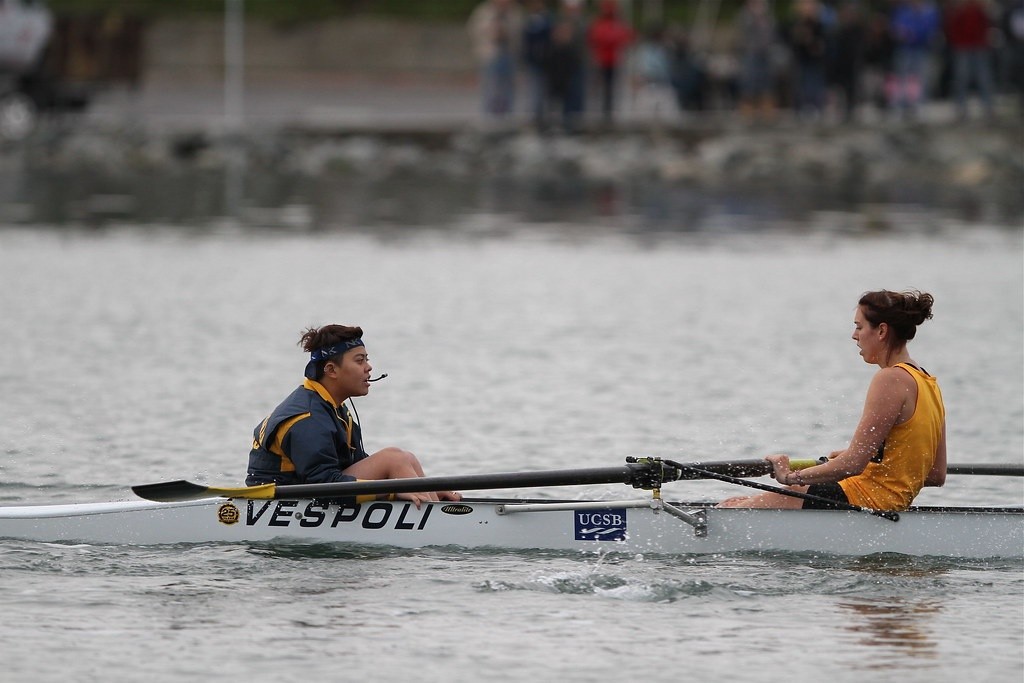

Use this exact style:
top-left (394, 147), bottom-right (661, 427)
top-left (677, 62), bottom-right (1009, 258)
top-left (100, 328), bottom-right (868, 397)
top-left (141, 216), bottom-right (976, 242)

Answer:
top-left (453, 492), bottom-right (456, 495)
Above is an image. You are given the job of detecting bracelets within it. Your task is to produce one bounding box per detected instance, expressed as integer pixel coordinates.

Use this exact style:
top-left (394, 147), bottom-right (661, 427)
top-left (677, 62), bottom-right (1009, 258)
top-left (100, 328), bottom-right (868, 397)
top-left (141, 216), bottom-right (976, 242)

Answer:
top-left (795, 470), bottom-right (804, 487)
top-left (387, 493), bottom-right (394, 500)
top-left (785, 471), bottom-right (793, 486)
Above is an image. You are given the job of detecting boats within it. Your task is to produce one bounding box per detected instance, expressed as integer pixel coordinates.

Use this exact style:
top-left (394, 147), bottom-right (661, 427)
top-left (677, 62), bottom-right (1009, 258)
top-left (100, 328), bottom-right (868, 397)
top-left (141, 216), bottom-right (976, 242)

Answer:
top-left (0, 498), bottom-right (1024, 573)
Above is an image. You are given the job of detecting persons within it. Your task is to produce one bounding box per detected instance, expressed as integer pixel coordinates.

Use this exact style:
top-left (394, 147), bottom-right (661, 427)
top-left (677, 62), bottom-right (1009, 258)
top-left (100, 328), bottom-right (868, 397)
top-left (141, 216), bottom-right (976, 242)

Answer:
top-left (466, 0), bottom-right (1024, 125)
top-left (716, 291), bottom-right (947, 514)
top-left (245, 325), bottom-right (463, 509)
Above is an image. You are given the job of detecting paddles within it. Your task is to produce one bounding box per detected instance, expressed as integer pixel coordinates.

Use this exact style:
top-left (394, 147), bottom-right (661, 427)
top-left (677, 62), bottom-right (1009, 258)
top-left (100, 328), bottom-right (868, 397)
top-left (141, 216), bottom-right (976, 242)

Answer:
top-left (132, 457), bottom-right (838, 503)
top-left (945, 462), bottom-right (1023, 477)
